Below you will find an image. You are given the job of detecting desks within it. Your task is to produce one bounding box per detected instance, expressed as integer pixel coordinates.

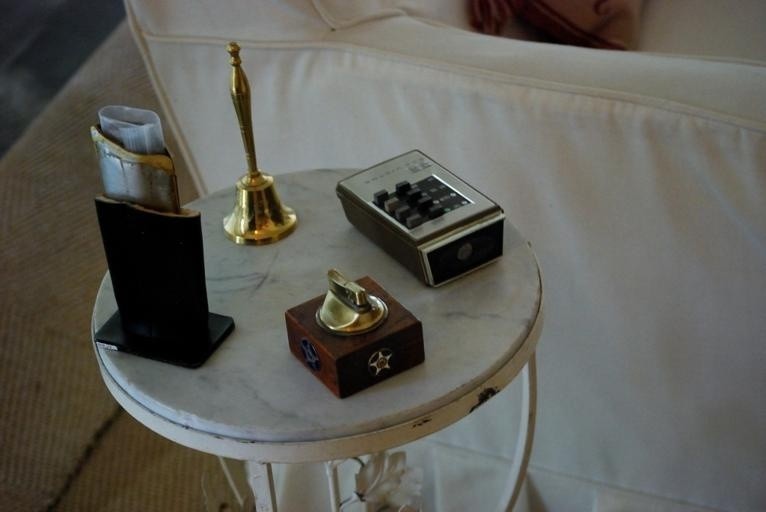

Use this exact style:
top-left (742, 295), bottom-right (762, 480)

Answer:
top-left (89, 164), bottom-right (547, 511)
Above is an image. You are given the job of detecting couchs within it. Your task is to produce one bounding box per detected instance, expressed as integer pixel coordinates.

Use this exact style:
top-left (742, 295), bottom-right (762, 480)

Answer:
top-left (125, 0), bottom-right (766, 512)
